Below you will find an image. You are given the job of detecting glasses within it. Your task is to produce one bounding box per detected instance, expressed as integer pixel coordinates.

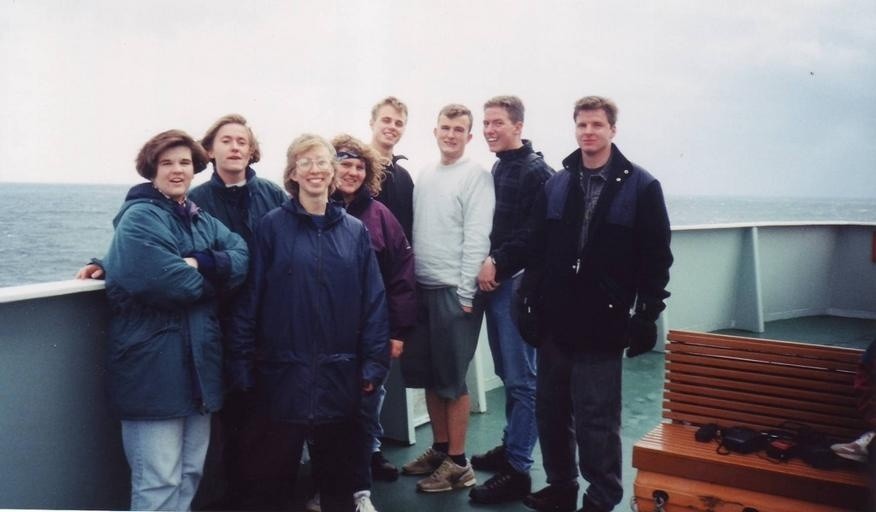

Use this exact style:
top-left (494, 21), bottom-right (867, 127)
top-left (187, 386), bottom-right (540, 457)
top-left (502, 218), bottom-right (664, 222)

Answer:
top-left (296, 158), bottom-right (333, 176)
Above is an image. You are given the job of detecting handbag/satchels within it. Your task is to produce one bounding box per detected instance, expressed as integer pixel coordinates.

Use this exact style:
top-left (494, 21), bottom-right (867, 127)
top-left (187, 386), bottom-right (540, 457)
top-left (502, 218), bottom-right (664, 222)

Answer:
top-left (695, 425), bottom-right (803, 465)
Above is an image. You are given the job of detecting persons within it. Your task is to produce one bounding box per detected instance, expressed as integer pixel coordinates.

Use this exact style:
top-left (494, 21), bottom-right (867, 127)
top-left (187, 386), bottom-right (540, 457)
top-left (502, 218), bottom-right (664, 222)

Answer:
top-left (403, 104), bottom-right (493, 492)
top-left (521, 96), bottom-right (673, 512)
top-left (74, 114), bottom-right (288, 511)
top-left (364, 97), bottom-right (428, 249)
top-left (468, 95), bottom-right (555, 503)
top-left (103, 129), bottom-right (251, 512)
top-left (229, 135), bottom-right (389, 512)
top-left (331, 133), bottom-right (419, 484)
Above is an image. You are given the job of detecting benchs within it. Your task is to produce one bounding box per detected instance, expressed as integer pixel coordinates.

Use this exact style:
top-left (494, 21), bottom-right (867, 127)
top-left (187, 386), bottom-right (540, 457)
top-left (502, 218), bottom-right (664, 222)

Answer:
top-left (630, 328), bottom-right (875, 512)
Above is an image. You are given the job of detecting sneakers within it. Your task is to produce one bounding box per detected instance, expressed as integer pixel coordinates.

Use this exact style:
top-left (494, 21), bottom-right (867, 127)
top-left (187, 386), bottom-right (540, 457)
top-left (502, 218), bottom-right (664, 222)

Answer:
top-left (578, 493), bottom-right (602, 511)
top-left (470, 447), bottom-right (510, 471)
top-left (417, 455), bottom-right (477, 493)
top-left (468, 462), bottom-right (532, 504)
top-left (831, 432), bottom-right (875, 463)
top-left (522, 485), bottom-right (577, 511)
top-left (402, 447), bottom-right (447, 475)
top-left (367, 451), bottom-right (399, 482)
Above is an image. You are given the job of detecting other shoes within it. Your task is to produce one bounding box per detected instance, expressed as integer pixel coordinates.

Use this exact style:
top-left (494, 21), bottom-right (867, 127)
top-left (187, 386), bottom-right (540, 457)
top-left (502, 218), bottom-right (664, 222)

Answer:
top-left (352, 489), bottom-right (376, 511)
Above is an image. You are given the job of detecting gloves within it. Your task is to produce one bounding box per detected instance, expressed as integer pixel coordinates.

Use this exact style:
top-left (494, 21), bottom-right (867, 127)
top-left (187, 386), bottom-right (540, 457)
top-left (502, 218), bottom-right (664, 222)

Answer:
top-left (516, 296), bottom-right (544, 348)
top-left (626, 313), bottom-right (657, 357)
